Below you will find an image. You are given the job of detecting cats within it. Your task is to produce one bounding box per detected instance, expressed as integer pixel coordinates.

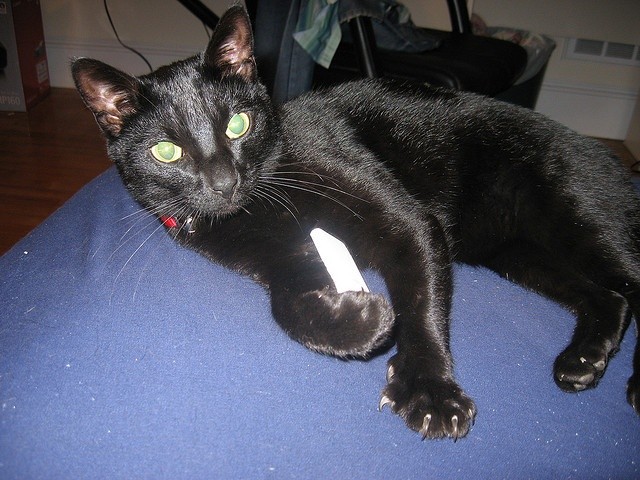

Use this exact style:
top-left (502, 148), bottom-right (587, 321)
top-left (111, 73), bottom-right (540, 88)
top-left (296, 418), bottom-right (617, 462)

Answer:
top-left (68, 3), bottom-right (639, 443)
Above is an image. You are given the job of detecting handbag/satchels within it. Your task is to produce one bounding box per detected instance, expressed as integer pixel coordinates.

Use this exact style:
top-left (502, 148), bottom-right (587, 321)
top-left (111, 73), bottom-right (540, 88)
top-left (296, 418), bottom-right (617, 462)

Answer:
top-left (309, 0), bottom-right (556, 120)
